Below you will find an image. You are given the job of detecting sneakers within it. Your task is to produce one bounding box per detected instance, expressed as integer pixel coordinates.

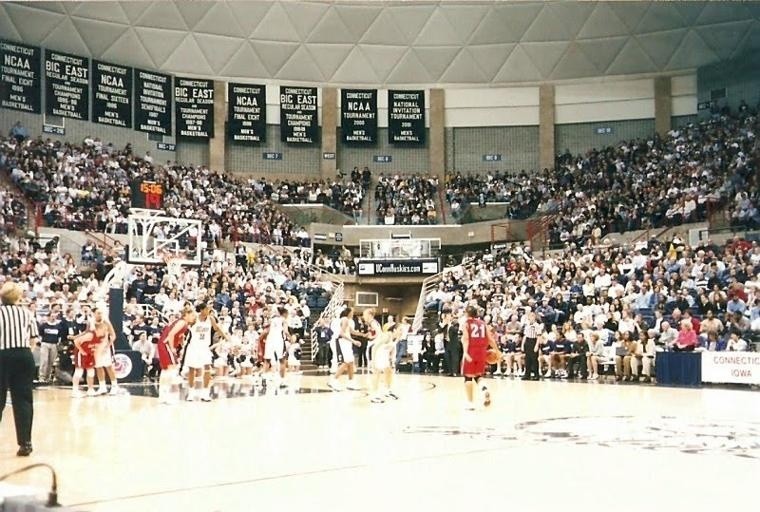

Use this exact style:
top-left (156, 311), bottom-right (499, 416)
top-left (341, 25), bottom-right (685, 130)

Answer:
top-left (326, 375), bottom-right (399, 404)
top-left (17, 440), bottom-right (32, 455)
top-left (185, 393), bottom-right (211, 401)
top-left (462, 388), bottom-right (491, 411)
top-left (70, 385), bottom-right (120, 397)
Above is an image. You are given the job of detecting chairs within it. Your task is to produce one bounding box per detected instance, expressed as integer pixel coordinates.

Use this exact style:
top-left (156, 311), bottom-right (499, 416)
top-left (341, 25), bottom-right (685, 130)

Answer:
top-left (81, 244), bottom-right (356, 367)
top-left (417, 305), bottom-right (760, 382)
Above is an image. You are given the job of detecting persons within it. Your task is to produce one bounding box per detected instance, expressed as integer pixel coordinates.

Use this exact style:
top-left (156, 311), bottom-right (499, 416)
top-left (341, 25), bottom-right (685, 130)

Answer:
top-left (1, 282), bottom-right (40, 455)
top-left (362, 98), bottom-right (759, 405)
top-left (1, 121), bottom-right (372, 400)
top-left (460, 305), bottom-right (501, 411)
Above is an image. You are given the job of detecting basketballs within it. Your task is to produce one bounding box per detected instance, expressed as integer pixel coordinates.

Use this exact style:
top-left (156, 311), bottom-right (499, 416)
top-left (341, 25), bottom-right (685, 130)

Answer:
top-left (486, 348), bottom-right (500, 366)
